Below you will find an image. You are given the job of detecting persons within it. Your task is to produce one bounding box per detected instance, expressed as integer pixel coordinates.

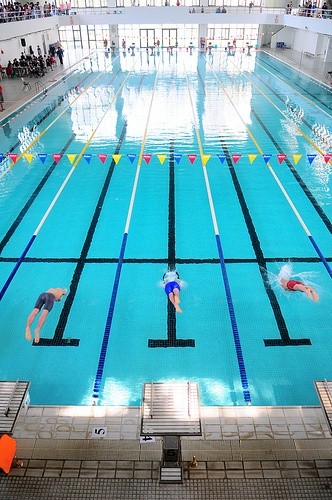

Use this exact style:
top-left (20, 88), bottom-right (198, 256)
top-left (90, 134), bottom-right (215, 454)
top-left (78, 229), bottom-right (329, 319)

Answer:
top-left (163, 269), bottom-right (184, 312)
top-left (0, 84), bottom-right (6, 112)
top-left (25, 288), bottom-right (68, 343)
top-left (280, 279), bottom-right (319, 302)
top-left (0, 0), bottom-right (332, 78)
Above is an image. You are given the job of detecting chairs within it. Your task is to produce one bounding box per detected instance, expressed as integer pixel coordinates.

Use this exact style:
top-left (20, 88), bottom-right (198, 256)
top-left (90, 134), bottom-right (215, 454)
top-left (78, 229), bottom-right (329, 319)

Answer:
top-left (21, 77), bottom-right (32, 90)
